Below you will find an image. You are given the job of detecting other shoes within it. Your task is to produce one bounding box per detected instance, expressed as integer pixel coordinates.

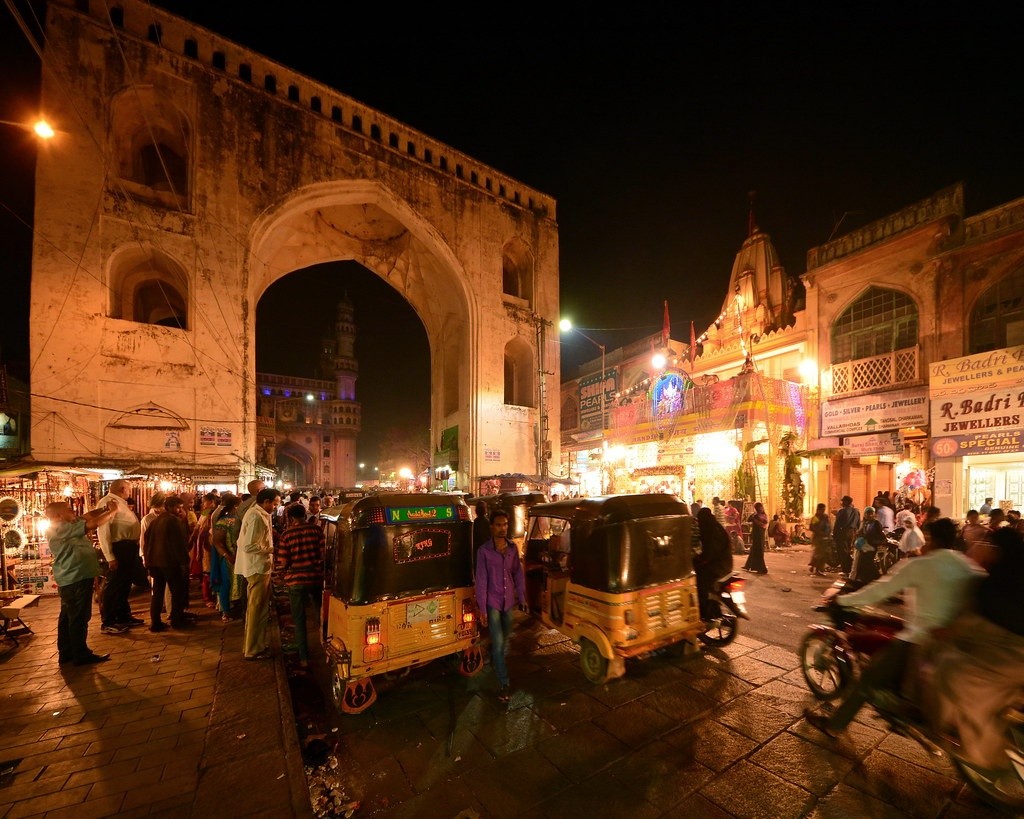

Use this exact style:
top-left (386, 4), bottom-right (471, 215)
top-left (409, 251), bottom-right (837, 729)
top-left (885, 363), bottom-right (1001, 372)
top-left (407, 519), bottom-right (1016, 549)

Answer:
top-left (814, 572), bottom-right (823, 576)
top-left (496, 685), bottom-right (510, 704)
top-left (171, 618), bottom-right (194, 627)
top-left (170, 612), bottom-right (194, 619)
top-left (756, 569), bottom-right (768, 576)
top-left (282, 643), bottom-right (299, 652)
top-left (222, 615), bottom-right (240, 623)
top-left (741, 566), bottom-right (750, 571)
top-left (151, 622), bottom-right (167, 632)
top-left (195, 575), bottom-right (199, 580)
top-left (809, 568), bottom-right (813, 572)
top-left (803, 708), bottom-right (849, 741)
top-left (205, 601), bottom-right (215, 609)
top-left (59, 649), bottom-right (93, 664)
top-left (189, 574), bottom-right (194, 579)
top-left (287, 661), bottom-right (309, 671)
top-left (72, 650), bottom-right (111, 665)
top-left (487, 640), bottom-right (496, 673)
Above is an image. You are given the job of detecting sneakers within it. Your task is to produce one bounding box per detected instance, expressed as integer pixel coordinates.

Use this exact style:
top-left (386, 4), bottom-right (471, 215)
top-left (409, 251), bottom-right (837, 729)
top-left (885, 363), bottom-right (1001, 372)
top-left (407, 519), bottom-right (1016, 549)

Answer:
top-left (101, 622), bottom-right (129, 634)
top-left (114, 614), bottom-right (144, 626)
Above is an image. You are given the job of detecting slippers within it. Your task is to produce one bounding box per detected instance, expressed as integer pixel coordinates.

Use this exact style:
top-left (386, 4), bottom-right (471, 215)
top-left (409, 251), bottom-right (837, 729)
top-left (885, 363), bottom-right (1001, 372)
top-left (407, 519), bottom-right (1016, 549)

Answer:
top-left (246, 652), bottom-right (271, 661)
top-left (243, 644), bottom-right (270, 656)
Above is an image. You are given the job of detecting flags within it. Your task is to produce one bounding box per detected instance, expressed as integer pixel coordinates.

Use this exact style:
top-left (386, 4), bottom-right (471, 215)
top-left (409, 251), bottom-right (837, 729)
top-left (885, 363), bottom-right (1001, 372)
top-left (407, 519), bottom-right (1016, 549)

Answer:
top-left (663, 303), bottom-right (670, 336)
top-left (691, 325), bottom-right (697, 359)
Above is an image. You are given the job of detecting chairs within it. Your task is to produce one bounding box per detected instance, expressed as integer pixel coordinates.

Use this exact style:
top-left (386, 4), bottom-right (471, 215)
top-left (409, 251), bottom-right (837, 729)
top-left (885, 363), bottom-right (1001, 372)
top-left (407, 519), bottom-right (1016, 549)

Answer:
top-left (326, 519), bottom-right (473, 665)
top-left (564, 514), bottom-right (693, 645)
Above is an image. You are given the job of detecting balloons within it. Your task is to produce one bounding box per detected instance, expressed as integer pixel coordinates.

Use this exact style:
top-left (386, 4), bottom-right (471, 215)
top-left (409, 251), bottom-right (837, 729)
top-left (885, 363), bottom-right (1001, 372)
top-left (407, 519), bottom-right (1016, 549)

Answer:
top-left (903, 472), bottom-right (925, 490)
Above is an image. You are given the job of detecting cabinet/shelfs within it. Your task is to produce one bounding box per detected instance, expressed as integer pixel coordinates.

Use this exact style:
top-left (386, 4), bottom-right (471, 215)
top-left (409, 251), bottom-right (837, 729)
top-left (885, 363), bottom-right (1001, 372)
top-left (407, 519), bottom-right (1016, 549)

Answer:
top-left (730, 501), bottom-right (754, 550)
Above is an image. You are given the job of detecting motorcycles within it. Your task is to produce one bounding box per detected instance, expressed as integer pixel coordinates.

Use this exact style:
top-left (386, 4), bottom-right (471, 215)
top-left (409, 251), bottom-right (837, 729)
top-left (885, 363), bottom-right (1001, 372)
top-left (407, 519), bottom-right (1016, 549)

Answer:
top-left (795, 572), bottom-right (1024, 819)
top-left (318, 489), bottom-right (487, 716)
top-left (463, 492), bottom-right (563, 601)
top-left (520, 494), bottom-right (709, 686)
top-left (695, 569), bottom-right (752, 647)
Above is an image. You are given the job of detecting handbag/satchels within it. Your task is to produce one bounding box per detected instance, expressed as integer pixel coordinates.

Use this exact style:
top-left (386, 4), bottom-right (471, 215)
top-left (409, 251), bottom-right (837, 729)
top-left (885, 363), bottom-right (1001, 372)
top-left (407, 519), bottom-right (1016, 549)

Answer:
top-left (132, 556), bottom-right (147, 586)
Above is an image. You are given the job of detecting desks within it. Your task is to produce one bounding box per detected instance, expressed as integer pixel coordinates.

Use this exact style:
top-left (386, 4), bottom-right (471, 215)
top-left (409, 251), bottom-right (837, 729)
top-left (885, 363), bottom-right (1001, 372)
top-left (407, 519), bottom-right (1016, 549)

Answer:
top-left (0, 595), bottom-right (41, 647)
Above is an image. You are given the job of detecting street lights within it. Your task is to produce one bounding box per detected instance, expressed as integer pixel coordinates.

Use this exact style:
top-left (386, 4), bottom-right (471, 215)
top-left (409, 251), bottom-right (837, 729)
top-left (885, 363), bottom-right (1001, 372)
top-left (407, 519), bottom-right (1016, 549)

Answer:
top-left (558, 318), bottom-right (606, 496)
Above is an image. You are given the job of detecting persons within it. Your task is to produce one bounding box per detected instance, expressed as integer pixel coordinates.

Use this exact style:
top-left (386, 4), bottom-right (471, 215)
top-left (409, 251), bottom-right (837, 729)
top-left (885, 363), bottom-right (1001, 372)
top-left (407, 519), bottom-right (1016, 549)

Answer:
top-left (0, 476), bottom-right (1024, 772)
top-left (741, 352), bottom-right (754, 373)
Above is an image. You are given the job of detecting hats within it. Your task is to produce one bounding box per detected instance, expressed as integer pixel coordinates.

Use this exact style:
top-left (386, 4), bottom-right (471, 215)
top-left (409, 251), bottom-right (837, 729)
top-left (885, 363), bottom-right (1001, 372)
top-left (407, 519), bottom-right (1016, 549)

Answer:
top-left (864, 506), bottom-right (875, 513)
top-left (841, 495), bottom-right (853, 504)
